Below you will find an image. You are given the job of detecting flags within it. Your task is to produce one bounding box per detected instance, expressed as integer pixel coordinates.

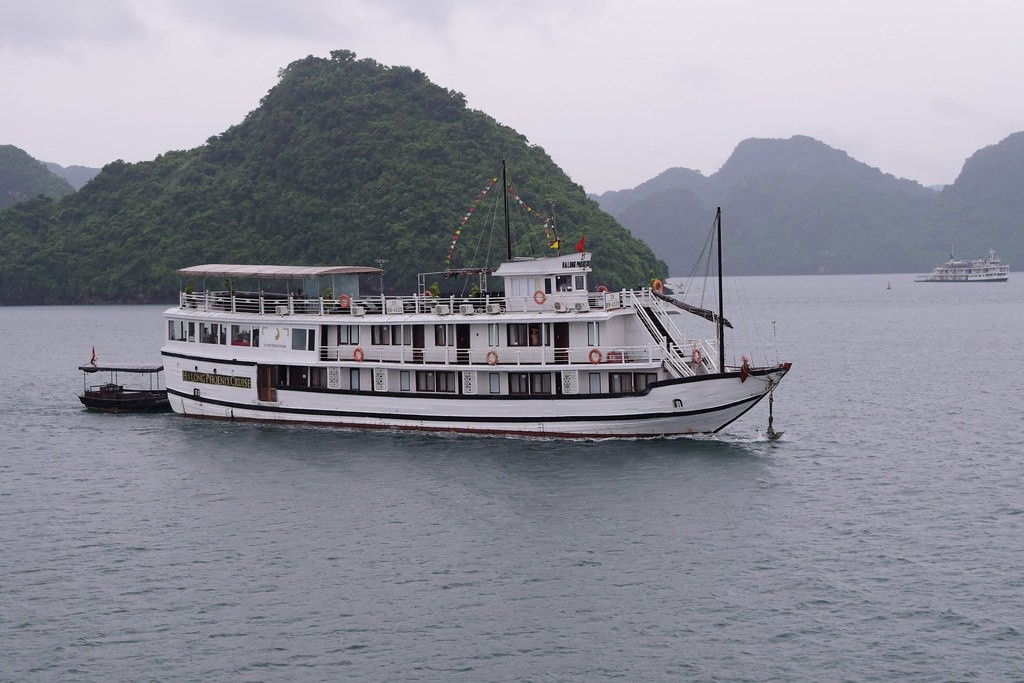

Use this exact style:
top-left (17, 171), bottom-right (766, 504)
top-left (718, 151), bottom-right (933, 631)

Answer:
top-left (550, 241), bottom-right (560, 250)
top-left (576, 237), bottom-right (586, 253)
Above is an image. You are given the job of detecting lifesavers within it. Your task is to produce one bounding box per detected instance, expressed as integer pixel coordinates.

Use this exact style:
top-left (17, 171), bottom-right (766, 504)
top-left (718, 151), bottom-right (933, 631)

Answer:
top-left (653, 279), bottom-right (663, 294)
top-left (423, 291), bottom-right (432, 296)
top-left (353, 347), bottom-right (364, 362)
top-left (339, 294), bottom-right (349, 308)
top-left (589, 349), bottom-right (602, 364)
top-left (533, 290), bottom-right (546, 305)
top-left (487, 351), bottom-right (498, 365)
top-left (596, 286), bottom-right (608, 292)
top-left (692, 349), bottom-right (701, 364)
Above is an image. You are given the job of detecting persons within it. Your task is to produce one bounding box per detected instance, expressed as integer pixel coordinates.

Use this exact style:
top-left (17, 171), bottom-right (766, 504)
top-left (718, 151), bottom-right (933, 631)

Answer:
top-left (559, 279), bottom-right (568, 292)
top-left (530, 328), bottom-right (540, 346)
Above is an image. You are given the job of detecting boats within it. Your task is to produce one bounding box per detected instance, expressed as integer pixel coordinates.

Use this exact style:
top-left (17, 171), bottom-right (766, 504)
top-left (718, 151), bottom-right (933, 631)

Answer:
top-left (76, 361), bottom-right (172, 414)
top-left (160, 159), bottom-right (793, 439)
top-left (913, 243), bottom-right (1010, 283)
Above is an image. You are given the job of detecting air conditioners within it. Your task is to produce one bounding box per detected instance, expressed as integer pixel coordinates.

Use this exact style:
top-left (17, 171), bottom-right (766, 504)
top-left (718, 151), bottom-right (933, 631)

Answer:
top-left (459, 305), bottom-right (473, 314)
top-left (276, 306), bottom-right (288, 314)
top-left (553, 301), bottom-right (566, 312)
top-left (351, 307), bottom-right (364, 315)
top-left (486, 304), bottom-right (500, 314)
top-left (573, 301), bottom-right (588, 312)
top-left (435, 304), bottom-right (449, 314)
